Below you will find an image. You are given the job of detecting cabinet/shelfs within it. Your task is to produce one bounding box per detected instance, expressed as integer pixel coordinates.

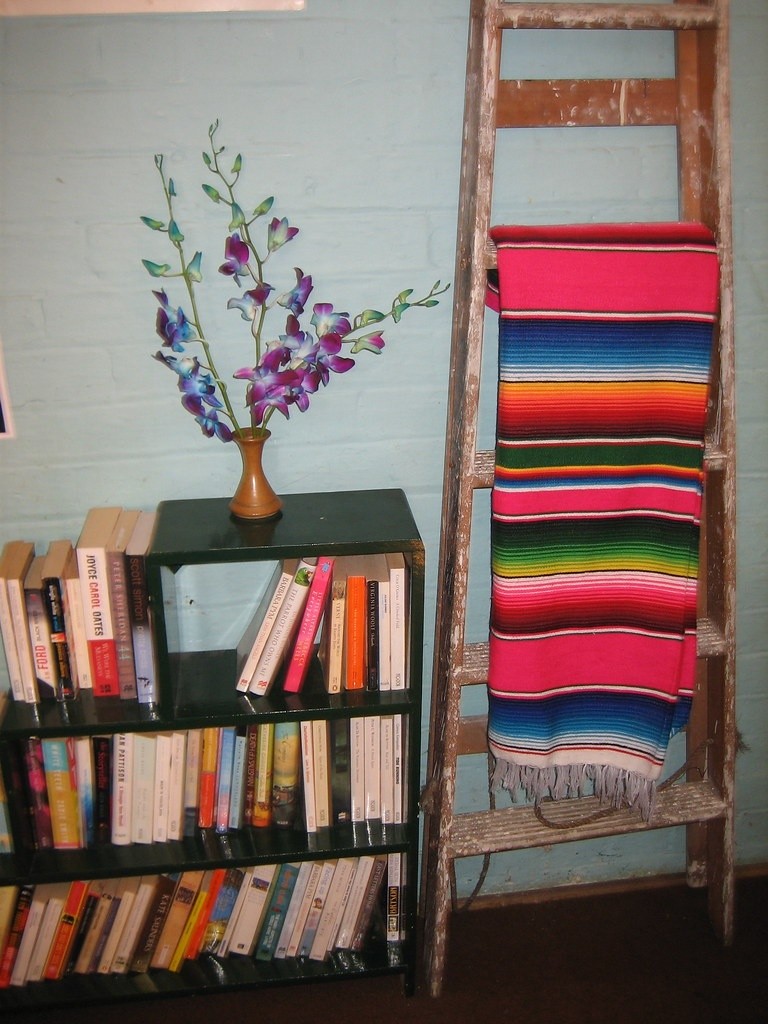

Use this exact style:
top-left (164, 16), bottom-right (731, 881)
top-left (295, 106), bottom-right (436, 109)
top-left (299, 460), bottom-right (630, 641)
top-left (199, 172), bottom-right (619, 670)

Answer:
top-left (0, 486), bottom-right (427, 1024)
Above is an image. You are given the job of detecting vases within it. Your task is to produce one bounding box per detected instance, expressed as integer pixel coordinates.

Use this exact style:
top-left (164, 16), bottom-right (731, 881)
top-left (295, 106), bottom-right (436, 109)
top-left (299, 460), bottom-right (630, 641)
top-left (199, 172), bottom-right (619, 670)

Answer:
top-left (228, 427), bottom-right (282, 519)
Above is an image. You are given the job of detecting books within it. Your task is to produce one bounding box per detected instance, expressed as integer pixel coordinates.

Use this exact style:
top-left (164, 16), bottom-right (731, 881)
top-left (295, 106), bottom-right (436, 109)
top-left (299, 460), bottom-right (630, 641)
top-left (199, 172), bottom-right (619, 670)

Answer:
top-left (0, 507), bottom-right (412, 989)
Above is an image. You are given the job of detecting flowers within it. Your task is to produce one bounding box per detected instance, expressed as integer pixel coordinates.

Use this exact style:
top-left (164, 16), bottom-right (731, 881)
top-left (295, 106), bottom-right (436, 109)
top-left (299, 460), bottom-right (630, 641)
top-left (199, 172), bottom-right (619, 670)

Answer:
top-left (138, 117), bottom-right (453, 443)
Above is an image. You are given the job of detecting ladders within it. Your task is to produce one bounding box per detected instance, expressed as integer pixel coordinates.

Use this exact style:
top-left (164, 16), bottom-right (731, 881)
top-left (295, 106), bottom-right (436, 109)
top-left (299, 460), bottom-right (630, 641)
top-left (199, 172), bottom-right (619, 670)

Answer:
top-left (416, 0), bottom-right (736, 1000)
